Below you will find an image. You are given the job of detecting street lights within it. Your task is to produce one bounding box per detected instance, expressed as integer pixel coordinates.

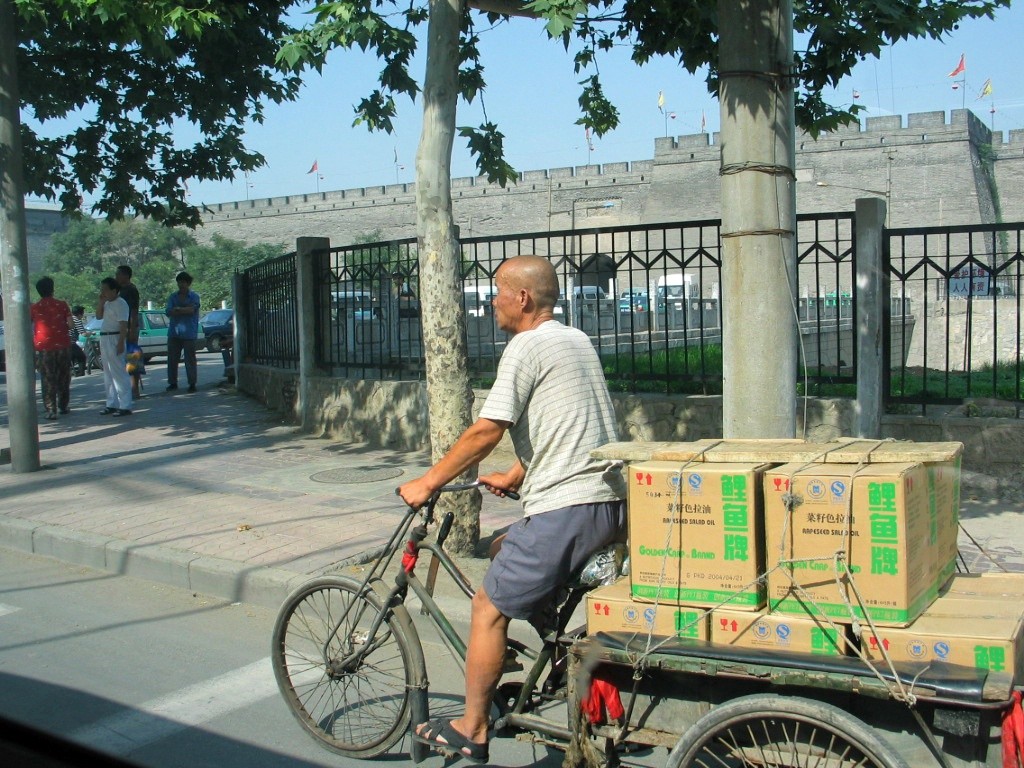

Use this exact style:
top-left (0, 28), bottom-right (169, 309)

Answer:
top-left (548, 203), bottom-right (614, 232)
top-left (817, 182), bottom-right (890, 229)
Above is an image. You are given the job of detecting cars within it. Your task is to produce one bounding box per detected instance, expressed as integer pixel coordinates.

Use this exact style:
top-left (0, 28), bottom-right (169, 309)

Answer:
top-left (76, 309), bottom-right (234, 368)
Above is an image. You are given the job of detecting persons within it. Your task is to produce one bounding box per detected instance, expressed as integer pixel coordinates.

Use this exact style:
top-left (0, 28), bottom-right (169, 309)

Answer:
top-left (397, 254), bottom-right (629, 766)
top-left (95, 278), bottom-right (132, 417)
top-left (29, 276), bottom-right (75, 420)
top-left (67, 306), bottom-right (96, 377)
top-left (115, 265), bottom-right (142, 400)
top-left (165, 272), bottom-right (201, 393)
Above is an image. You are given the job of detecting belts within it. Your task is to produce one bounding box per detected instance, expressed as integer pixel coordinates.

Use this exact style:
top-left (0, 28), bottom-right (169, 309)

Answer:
top-left (100, 332), bottom-right (120, 336)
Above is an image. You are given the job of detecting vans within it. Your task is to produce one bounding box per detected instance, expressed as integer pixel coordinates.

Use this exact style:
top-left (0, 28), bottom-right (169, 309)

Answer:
top-left (657, 274), bottom-right (699, 310)
top-left (554, 286), bottom-right (647, 314)
top-left (464, 286), bottom-right (498, 317)
top-left (330, 292), bottom-right (386, 320)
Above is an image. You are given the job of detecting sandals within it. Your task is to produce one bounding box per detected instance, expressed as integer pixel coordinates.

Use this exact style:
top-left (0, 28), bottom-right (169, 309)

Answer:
top-left (114, 408), bottom-right (131, 416)
top-left (100, 407), bottom-right (115, 415)
top-left (412, 718), bottom-right (490, 763)
top-left (542, 665), bottom-right (570, 699)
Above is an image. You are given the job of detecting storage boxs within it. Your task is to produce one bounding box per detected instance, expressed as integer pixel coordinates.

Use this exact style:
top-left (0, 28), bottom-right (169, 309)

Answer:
top-left (585, 437), bottom-right (1023, 686)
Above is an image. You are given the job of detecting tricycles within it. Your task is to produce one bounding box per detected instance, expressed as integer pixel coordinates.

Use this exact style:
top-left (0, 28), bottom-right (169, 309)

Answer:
top-left (71, 332), bottom-right (103, 376)
top-left (272, 479), bottom-right (1024, 768)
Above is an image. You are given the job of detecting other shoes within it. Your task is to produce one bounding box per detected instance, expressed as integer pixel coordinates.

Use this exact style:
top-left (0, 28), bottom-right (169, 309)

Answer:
top-left (60, 407), bottom-right (70, 414)
top-left (44, 412), bottom-right (56, 420)
top-left (166, 383), bottom-right (177, 392)
top-left (189, 384), bottom-right (196, 393)
